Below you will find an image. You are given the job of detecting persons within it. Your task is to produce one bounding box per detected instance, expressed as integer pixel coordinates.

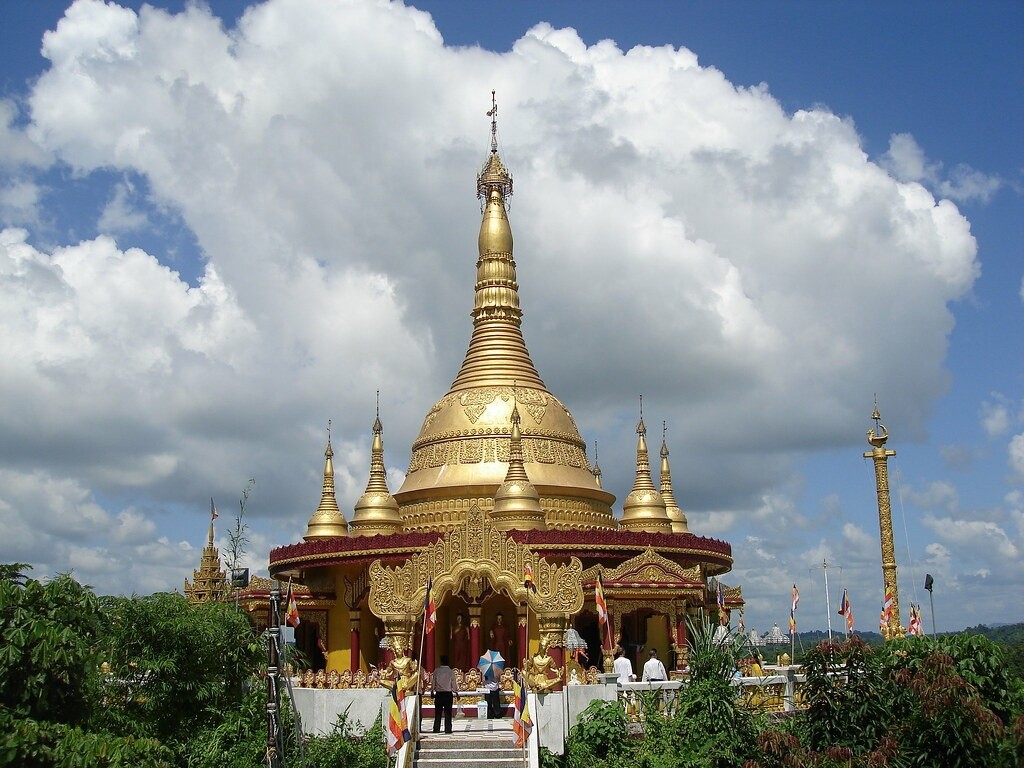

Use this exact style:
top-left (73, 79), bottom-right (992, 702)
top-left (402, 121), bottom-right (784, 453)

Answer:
top-left (520, 632), bottom-right (563, 695)
top-left (450, 612), bottom-right (470, 670)
top-left (295, 668), bottom-right (517, 691)
top-left (718, 653), bottom-right (764, 705)
top-left (488, 612), bottom-right (513, 668)
top-left (566, 668), bottom-right (582, 686)
top-left (641, 648), bottom-right (668, 711)
top-left (613, 647), bottom-right (637, 713)
top-left (577, 648), bottom-right (589, 670)
top-left (412, 655), bottom-right (460, 734)
top-left (484, 671), bottom-right (503, 719)
top-left (379, 635), bottom-right (419, 696)
top-left (586, 666), bottom-right (601, 684)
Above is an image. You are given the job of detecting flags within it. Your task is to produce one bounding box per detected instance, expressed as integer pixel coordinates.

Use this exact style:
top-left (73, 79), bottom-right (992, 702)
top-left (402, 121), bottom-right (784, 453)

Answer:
top-left (524, 564), bottom-right (538, 593)
top-left (385, 671), bottom-right (413, 760)
top-left (594, 569), bottom-right (608, 624)
top-left (284, 574), bottom-right (300, 628)
top-left (210, 497), bottom-right (219, 521)
top-left (422, 575), bottom-right (437, 635)
top-left (715, 579), bottom-right (923, 638)
top-left (511, 667), bottom-right (535, 748)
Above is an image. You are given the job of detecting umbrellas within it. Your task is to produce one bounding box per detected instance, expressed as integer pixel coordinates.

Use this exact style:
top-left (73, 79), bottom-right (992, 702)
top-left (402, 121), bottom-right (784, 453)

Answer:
top-left (477, 649), bottom-right (505, 679)
